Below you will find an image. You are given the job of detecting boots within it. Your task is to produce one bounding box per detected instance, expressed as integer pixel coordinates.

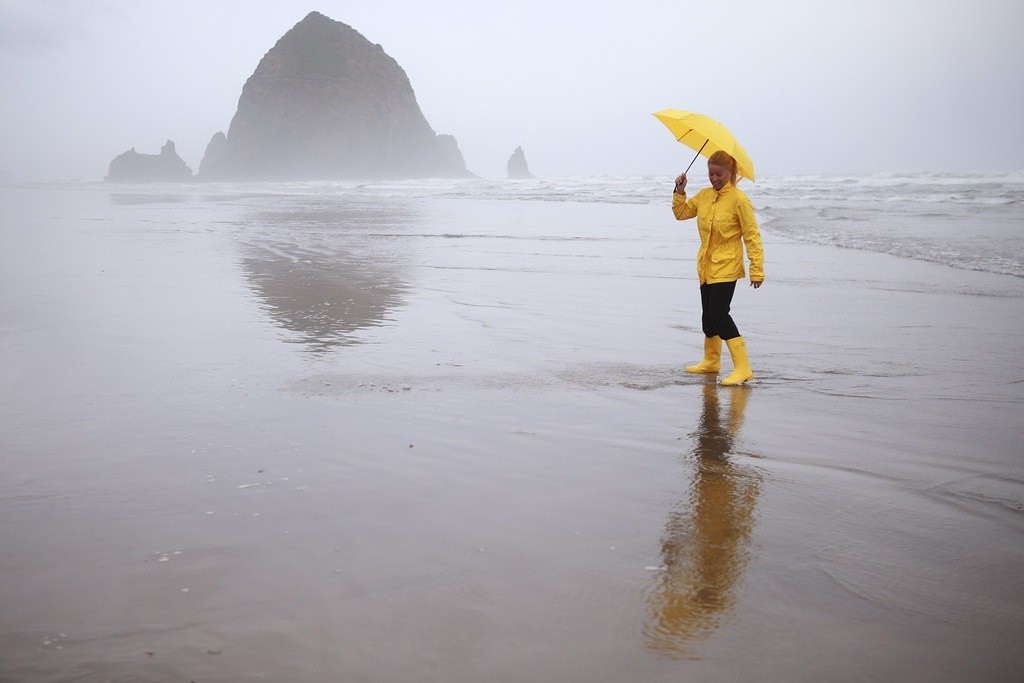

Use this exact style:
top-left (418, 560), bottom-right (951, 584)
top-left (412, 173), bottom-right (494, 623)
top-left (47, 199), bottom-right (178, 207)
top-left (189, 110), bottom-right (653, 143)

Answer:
top-left (687, 334), bottom-right (722, 374)
top-left (719, 335), bottom-right (753, 385)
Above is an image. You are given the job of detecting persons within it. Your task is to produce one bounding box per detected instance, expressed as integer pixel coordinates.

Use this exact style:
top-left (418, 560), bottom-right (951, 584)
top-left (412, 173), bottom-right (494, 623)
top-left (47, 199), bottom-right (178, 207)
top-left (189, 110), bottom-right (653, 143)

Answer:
top-left (672, 151), bottom-right (765, 386)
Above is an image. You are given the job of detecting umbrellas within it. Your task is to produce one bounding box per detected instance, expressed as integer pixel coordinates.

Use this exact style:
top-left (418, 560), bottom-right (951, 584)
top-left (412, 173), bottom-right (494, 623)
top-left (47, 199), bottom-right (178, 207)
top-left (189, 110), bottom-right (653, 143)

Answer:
top-left (652, 110), bottom-right (756, 187)
